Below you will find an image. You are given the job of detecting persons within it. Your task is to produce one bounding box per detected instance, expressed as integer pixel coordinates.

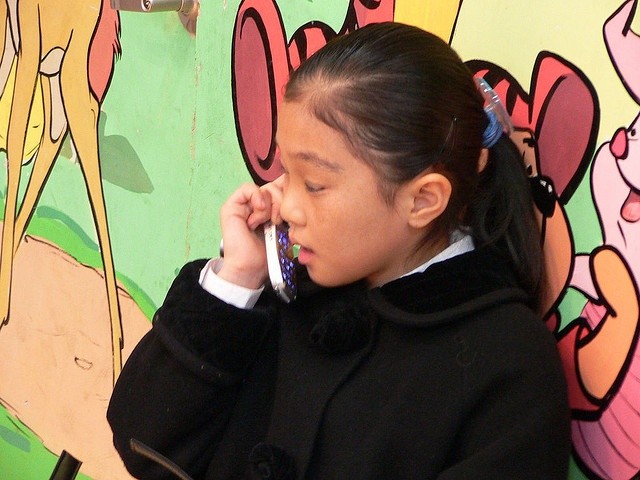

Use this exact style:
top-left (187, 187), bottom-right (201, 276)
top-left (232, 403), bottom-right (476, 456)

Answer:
top-left (107, 22), bottom-right (571, 480)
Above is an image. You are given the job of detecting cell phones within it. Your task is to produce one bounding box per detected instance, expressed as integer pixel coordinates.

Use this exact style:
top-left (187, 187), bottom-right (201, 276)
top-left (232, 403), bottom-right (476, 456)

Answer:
top-left (263, 221), bottom-right (298, 305)
